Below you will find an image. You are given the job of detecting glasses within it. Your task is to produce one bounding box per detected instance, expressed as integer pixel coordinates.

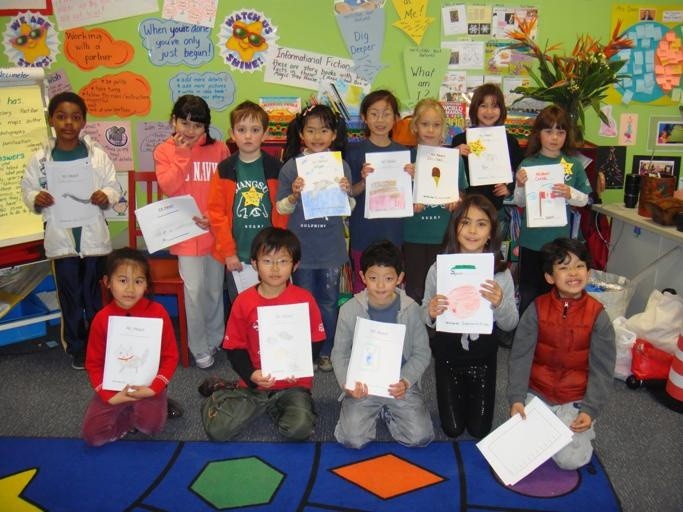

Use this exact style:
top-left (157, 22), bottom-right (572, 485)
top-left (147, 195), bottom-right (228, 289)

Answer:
top-left (263, 257), bottom-right (290, 266)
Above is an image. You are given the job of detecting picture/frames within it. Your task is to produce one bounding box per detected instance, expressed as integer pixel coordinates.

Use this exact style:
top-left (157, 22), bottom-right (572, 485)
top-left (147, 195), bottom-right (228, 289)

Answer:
top-left (633, 154), bottom-right (682, 192)
top-left (657, 121), bottom-right (683, 146)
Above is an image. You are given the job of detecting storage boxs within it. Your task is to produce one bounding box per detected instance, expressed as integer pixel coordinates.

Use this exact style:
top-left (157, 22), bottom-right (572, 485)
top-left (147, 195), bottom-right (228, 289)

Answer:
top-left (26, 275), bottom-right (61, 327)
top-left (0, 293), bottom-right (47, 347)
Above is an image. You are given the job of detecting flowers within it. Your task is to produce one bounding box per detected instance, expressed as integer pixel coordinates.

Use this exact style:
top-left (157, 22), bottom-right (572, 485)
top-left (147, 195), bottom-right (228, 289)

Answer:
top-left (495, 14), bottom-right (635, 131)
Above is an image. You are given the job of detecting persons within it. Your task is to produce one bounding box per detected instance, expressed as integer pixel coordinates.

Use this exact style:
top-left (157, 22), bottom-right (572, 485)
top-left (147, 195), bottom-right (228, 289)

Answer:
top-left (274, 103), bottom-right (353, 370)
top-left (154, 95), bottom-right (230, 369)
top-left (197, 228), bottom-right (327, 443)
top-left (421, 193), bottom-right (517, 437)
top-left (452, 85), bottom-right (520, 264)
top-left (514, 105), bottom-right (591, 318)
top-left (346, 90), bottom-right (413, 291)
top-left (401, 99), bottom-right (467, 306)
top-left (21, 91), bottom-right (120, 369)
top-left (205, 101), bottom-right (284, 302)
top-left (329, 241), bottom-right (434, 449)
top-left (79, 248), bottom-right (183, 449)
top-left (507, 238), bottom-right (615, 470)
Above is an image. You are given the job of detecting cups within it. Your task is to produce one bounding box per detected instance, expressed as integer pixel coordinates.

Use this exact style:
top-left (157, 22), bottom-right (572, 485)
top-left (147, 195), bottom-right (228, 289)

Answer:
top-left (623, 173), bottom-right (639, 208)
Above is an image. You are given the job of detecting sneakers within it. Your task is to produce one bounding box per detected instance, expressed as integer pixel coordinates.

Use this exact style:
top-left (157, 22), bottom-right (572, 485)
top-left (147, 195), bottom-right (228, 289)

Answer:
top-left (318, 355), bottom-right (333, 372)
top-left (192, 346), bottom-right (218, 368)
top-left (199, 377), bottom-right (238, 396)
top-left (167, 399), bottom-right (183, 418)
top-left (72, 352), bottom-right (85, 369)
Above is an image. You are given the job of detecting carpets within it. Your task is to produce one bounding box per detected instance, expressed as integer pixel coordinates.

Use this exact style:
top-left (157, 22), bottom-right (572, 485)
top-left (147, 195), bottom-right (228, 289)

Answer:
top-left (0, 436), bottom-right (623, 512)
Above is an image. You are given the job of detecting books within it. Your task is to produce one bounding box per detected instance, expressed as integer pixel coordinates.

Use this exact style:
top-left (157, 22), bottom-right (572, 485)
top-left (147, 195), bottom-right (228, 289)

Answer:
top-left (307, 81), bottom-right (351, 123)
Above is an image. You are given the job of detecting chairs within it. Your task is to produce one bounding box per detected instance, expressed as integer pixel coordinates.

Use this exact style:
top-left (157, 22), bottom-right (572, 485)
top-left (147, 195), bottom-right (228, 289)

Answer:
top-left (99, 169), bottom-right (190, 368)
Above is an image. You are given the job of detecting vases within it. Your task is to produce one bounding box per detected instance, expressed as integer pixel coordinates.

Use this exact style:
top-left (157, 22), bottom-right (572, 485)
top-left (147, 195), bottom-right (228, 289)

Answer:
top-left (555, 99), bottom-right (586, 146)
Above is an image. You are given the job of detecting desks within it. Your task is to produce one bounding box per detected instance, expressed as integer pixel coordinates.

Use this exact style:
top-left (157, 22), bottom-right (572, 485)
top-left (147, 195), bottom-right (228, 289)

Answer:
top-left (590, 201), bottom-right (683, 319)
top-left (515, 138), bottom-right (600, 191)
